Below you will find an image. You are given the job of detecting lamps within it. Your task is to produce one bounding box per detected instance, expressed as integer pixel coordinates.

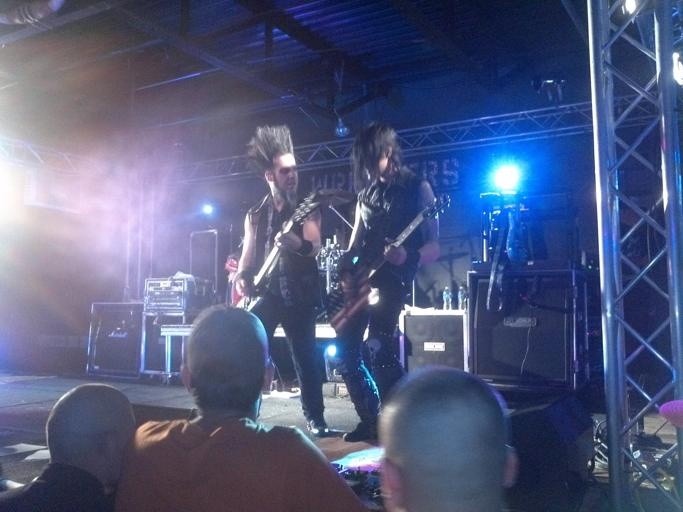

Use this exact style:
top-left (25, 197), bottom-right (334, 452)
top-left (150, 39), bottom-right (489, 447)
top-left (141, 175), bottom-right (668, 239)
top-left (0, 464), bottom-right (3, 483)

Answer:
top-left (331, 106), bottom-right (350, 138)
top-left (530, 72), bottom-right (567, 111)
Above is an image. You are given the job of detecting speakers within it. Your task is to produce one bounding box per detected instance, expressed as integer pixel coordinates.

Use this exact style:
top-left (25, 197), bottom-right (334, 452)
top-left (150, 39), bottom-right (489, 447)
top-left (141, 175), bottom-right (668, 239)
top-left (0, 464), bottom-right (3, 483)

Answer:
top-left (89, 303), bottom-right (142, 380)
top-left (473, 271), bottom-right (570, 385)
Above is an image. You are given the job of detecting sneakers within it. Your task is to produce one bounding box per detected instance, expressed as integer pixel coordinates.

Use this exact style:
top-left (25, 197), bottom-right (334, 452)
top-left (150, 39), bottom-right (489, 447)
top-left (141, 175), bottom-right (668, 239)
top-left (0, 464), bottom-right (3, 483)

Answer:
top-left (341, 418), bottom-right (378, 441)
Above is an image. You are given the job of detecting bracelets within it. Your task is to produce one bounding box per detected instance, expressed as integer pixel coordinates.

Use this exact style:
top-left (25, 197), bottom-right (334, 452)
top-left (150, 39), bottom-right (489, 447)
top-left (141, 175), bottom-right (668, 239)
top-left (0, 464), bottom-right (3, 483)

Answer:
top-left (404, 246), bottom-right (421, 266)
top-left (296, 239), bottom-right (313, 257)
top-left (235, 271), bottom-right (252, 282)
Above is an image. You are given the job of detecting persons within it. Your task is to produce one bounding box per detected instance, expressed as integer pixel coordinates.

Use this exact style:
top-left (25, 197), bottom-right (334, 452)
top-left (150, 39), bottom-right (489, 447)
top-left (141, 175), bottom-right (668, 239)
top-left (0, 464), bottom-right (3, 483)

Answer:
top-left (225, 252), bottom-right (242, 305)
top-left (114, 306), bottom-right (368, 511)
top-left (0, 384), bottom-right (136, 512)
top-left (334, 121), bottom-right (440, 442)
top-left (377, 365), bottom-right (520, 512)
top-left (234, 125), bottom-right (331, 438)
top-left (442, 286), bottom-right (452, 310)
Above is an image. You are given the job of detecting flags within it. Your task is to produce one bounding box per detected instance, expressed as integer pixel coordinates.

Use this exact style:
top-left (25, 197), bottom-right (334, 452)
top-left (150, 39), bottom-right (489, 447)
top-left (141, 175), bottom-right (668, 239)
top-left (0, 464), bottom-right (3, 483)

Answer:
top-left (325, 258), bottom-right (371, 334)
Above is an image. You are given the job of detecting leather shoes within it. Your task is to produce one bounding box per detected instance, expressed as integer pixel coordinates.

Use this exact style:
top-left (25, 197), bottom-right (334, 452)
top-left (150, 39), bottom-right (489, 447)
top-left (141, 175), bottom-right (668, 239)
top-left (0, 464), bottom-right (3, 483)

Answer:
top-left (305, 414), bottom-right (331, 438)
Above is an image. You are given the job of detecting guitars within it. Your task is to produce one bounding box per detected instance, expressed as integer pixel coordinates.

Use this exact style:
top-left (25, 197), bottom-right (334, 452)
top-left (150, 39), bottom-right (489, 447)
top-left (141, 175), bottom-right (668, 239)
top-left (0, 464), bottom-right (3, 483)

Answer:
top-left (232, 189), bottom-right (323, 315)
top-left (324, 195), bottom-right (450, 336)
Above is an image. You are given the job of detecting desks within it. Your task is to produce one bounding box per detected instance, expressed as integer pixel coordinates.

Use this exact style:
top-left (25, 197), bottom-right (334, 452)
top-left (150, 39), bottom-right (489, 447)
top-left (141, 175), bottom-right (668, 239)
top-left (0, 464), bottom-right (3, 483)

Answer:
top-left (161, 324), bottom-right (337, 387)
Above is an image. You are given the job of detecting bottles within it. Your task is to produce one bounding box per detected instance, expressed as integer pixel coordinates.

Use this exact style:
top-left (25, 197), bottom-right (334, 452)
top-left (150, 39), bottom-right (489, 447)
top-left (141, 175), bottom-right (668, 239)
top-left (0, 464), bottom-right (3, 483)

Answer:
top-left (441, 286), bottom-right (453, 310)
